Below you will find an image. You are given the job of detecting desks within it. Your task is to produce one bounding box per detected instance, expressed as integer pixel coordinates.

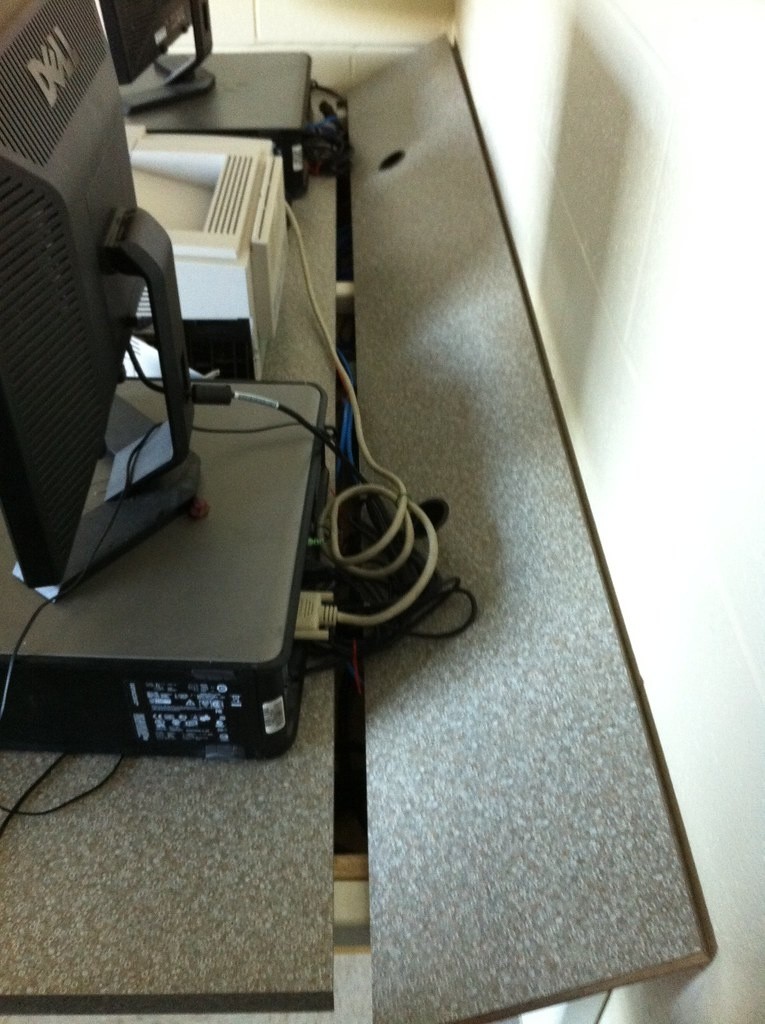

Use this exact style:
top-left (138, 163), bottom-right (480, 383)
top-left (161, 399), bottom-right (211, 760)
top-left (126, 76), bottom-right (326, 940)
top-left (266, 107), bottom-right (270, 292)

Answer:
top-left (0, 173), bottom-right (337, 1018)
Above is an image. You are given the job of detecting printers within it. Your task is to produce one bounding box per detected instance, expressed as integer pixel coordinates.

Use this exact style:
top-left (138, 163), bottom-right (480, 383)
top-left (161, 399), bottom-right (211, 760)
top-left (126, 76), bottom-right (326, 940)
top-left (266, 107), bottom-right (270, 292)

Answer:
top-left (114, 125), bottom-right (290, 384)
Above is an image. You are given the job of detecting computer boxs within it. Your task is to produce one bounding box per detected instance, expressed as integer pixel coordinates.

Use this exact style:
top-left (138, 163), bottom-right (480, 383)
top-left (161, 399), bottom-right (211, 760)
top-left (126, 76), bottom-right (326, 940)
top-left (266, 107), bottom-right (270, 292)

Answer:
top-left (120, 52), bottom-right (314, 199)
top-left (0, 375), bottom-right (329, 760)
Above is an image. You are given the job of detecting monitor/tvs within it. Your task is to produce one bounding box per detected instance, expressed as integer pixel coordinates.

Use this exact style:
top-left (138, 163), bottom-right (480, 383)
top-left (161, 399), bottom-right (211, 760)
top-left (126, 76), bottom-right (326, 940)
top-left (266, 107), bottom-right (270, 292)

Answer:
top-left (0, 0), bottom-right (203, 604)
top-left (98, 0), bottom-right (216, 116)
